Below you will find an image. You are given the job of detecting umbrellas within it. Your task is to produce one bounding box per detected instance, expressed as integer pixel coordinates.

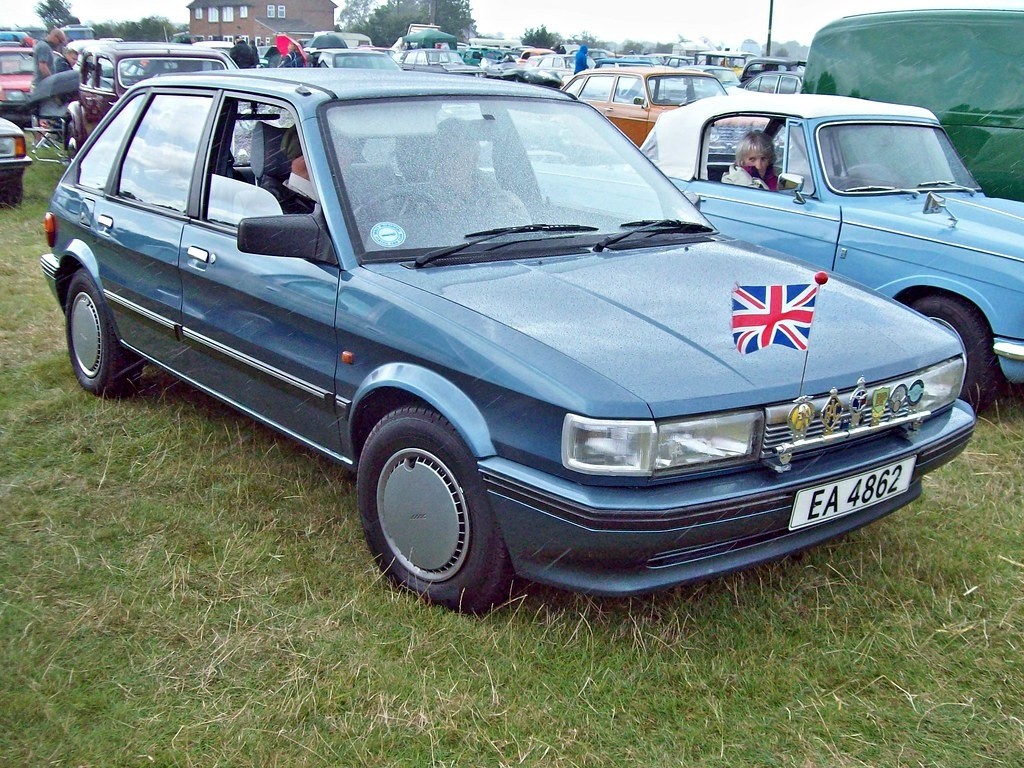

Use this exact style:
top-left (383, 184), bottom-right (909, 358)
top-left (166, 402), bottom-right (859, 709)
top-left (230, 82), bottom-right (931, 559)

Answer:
top-left (307, 32), bottom-right (349, 49)
top-left (274, 33), bottom-right (308, 63)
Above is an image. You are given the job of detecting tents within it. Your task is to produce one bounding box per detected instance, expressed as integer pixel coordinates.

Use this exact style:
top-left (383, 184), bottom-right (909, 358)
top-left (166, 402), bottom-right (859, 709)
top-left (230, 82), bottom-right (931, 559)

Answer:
top-left (400, 28), bottom-right (459, 52)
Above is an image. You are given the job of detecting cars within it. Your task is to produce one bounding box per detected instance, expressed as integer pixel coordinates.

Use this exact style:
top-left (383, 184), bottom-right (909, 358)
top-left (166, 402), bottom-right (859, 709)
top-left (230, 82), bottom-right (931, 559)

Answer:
top-left (0, 47), bottom-right (37, 122)
top-left (36, 23), bottom-right (811, 179)
top-left (39, 67), bottom-right (980, 616)
top-left (0, 114), bottom-right (35, 206)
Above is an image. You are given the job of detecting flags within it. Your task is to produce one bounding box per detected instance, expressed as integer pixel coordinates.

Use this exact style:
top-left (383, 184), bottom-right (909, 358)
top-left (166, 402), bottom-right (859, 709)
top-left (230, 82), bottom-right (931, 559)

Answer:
top-left (730, 282), bottom-right (819, 353)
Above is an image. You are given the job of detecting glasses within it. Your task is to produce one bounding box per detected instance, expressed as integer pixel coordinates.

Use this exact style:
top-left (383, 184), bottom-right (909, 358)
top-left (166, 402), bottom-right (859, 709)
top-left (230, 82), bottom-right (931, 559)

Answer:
top-left (56, 36), bottom-right (62, 43)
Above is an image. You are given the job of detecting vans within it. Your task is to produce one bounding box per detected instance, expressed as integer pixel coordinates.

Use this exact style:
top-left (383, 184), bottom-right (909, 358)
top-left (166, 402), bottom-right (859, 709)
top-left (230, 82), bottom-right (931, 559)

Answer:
top-left (799, 7), bottom-right (1024, 205)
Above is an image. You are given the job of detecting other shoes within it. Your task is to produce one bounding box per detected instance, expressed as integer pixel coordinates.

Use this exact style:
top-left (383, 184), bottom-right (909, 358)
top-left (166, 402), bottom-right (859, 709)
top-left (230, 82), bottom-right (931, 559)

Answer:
top-left (32, 141), bottom-right (50, 148)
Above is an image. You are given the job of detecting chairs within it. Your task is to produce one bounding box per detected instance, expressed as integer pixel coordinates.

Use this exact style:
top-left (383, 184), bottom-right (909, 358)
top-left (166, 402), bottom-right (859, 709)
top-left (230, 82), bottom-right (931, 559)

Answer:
top-left (342, 160), bottom-right (394, 224)
top-left (250, 121), bottom-right (299, 211)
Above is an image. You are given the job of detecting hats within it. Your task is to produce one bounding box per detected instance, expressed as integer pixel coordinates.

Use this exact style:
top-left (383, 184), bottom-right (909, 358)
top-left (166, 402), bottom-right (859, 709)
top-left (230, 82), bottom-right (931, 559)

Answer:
top-left (233, 34), bottom-right (242, 44)
top-left (281, 124), bottom-right (303, 162)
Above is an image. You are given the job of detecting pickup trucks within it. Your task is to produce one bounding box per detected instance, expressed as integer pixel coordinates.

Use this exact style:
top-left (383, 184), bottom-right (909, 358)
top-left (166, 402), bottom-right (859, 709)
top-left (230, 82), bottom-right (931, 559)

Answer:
top-left (470, 87), bottom-right (1024, 418)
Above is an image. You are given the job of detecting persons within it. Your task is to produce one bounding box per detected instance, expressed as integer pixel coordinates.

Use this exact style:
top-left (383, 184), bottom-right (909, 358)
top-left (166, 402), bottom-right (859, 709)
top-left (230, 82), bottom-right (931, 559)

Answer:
top-left (54, 48), bottom-right (81, 144)
top-left (261, 118), bottom-right (357, 262)
top-left (721, 130), bottom-right (779, 191)
top-left (435, 43), bottom-right (442, 49)
top-left (31, 28), bottom-right (67, 149)
top-left (574, 45), bottom-right (588, 76)
top-left (418, 118), bottom-right (533, 243)
top-left (406, 41), bottom-right (413, 50)
top-left (230, 33), bottom-right (313, 67)
